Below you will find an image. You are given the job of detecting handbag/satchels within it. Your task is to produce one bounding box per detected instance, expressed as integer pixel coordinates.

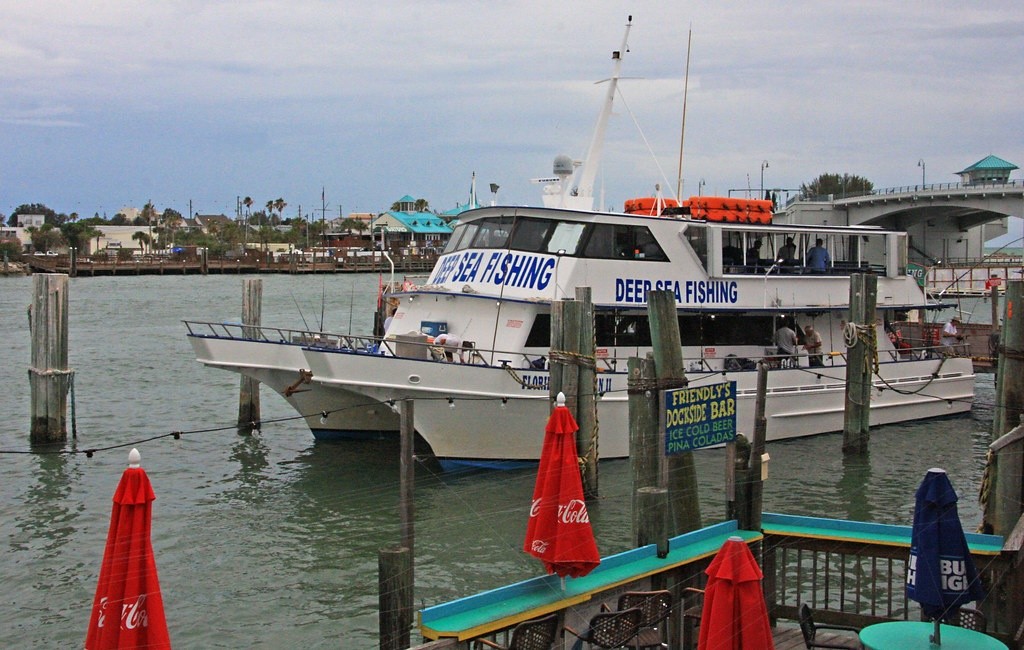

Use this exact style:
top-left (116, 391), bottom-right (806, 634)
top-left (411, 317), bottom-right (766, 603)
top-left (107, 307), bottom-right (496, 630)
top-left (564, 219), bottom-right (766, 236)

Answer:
top-left (741, 357), bottom-right (757, 369)
top-left (724, 354), bottom-right (742, 371)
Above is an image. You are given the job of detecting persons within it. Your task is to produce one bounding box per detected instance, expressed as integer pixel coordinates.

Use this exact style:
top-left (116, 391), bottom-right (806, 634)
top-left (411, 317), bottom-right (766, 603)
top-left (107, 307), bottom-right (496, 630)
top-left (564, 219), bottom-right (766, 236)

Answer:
top-left (775, 319), bottom-right (798, 368)
top-left (742, 237), bottom-right (829, 276)
top-left (801, 326), bottom-right (824, 366)
top-left (939, 315), bottom-right (964, 353)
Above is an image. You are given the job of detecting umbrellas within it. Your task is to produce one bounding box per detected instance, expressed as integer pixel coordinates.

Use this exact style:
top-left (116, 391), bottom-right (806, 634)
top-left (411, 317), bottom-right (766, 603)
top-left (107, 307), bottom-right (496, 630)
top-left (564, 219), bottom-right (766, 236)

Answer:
top-left (905, 467), bottom-right (982, 650)
top-left (696, 536), bottom-right (773, 650)
top-left (84, 446), bottom-right (173, 650)
top-left (523, 392), bottom-right (600, 592)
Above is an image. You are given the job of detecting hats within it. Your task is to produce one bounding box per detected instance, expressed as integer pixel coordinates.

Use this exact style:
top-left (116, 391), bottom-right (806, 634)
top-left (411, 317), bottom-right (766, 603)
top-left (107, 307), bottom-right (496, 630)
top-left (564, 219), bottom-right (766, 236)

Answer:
top-left (754, 240), bottom-right (763, 245)
top-left (950, 316), bottom-right (961, 323)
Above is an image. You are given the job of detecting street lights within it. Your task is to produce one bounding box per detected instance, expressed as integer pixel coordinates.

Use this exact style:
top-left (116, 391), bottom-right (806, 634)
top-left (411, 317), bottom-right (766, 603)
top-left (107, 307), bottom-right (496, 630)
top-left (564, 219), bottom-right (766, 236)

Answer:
top-left (760, 160), bottom-right (769, 200)
top-left (699, 178), bottom-right (706, 196)
top-left (917, 158), bottom-right (925, 186)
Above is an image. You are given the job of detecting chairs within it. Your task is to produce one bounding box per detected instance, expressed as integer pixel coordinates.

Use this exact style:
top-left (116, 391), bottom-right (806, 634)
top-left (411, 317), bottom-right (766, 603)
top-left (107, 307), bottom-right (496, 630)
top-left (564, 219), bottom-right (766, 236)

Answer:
top-left (798, 603), bottom-right (865, 650)
top-left (396, 587), bottom-right (706, 650)
top-left (947, 607), bottom-right (988, 633)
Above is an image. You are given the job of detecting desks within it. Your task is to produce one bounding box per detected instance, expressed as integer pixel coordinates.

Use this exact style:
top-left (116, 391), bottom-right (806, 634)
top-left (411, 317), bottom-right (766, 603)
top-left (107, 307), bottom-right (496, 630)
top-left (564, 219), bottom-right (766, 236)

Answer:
top-left (859, 621), bottom-right (1010, 650)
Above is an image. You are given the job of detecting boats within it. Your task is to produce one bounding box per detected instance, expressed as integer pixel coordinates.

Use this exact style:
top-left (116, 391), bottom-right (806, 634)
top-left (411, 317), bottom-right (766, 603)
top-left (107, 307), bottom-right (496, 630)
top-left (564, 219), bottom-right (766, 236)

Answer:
top-left (181, 16), bottom-right (977, 475)
